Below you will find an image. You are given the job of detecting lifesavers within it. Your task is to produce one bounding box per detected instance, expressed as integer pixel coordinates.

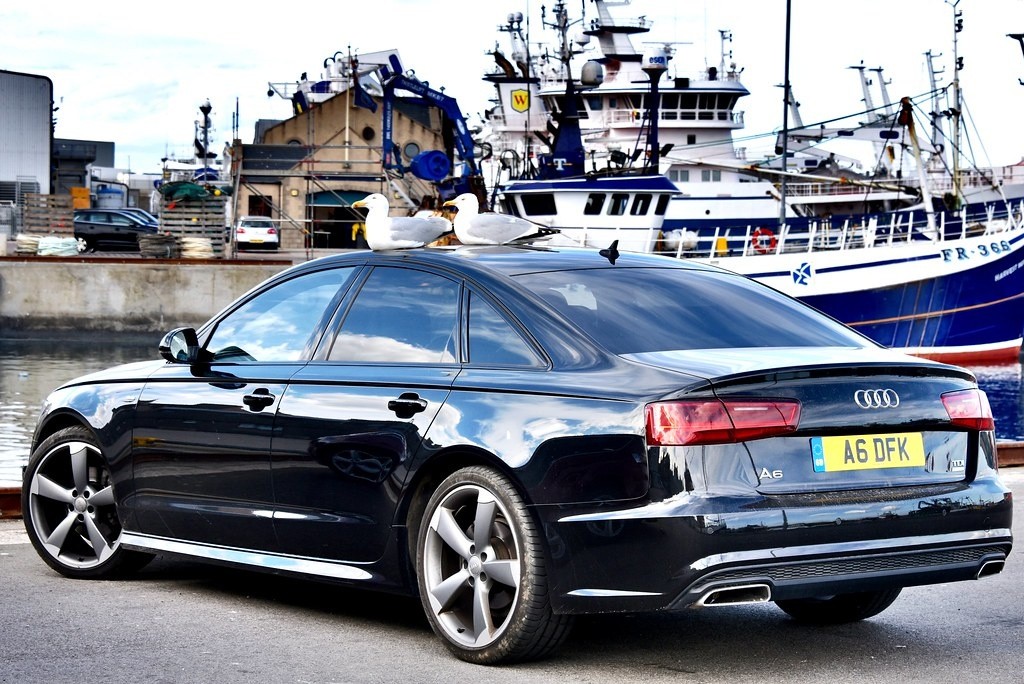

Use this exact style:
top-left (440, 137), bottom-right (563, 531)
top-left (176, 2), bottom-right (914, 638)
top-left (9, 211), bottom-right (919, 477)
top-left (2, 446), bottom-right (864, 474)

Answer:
top-left (752, 229), bottom-right (776, 253)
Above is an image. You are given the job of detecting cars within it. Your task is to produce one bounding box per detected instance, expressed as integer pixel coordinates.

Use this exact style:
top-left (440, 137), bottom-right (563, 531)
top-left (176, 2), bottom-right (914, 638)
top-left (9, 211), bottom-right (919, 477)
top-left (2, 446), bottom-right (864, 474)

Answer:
top-left (18, 240), bottom-right (1015, 669)
top-left (234, 215), bottom-right (279, 252)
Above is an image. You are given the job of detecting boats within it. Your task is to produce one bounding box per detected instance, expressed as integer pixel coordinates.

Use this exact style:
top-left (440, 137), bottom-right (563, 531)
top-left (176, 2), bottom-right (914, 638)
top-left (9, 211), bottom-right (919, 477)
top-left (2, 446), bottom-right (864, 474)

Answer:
top-left (489, 167), bottom-right (1024, 370)
top-left (481, 0), bottom-right (1024, 256)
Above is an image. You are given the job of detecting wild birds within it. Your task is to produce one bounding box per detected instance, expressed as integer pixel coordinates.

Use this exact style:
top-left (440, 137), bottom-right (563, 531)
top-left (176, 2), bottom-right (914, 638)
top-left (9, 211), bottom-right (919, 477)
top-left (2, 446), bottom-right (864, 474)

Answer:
top-left (443, 193), bottom-right (561, 245)
top-left (350, 193), bottom-right (453, 250)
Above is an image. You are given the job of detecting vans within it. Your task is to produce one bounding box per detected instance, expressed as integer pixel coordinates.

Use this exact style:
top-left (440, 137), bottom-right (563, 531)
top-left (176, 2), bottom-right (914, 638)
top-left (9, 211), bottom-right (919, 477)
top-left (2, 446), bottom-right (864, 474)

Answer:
top-left (60, 208), bottom-right (177, 254)
